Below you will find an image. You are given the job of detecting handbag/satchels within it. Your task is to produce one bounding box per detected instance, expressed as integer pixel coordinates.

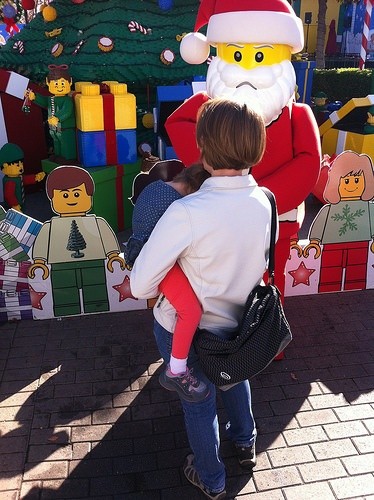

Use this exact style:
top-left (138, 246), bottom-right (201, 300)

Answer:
top-left (192, 282), bottom-right (292, 386)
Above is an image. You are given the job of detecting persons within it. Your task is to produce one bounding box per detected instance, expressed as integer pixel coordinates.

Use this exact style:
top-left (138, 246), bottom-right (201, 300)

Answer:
top-left (0, 142), bottom-right (46, 213)
top-left (22, 66), bottom-right (77, 165)
top-left (131, 99), bottom-right (280, 499)
top-left (125, 164), bottom-right (211, 403)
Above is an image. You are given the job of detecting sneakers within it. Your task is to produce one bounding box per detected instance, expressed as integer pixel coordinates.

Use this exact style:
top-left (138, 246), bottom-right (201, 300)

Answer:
top-left (158, 364), bottom-right (210, 403)
top-left (235, 442), bottom-right (256, 468)
top-left (183, 454), bottom-right (226, 500)
